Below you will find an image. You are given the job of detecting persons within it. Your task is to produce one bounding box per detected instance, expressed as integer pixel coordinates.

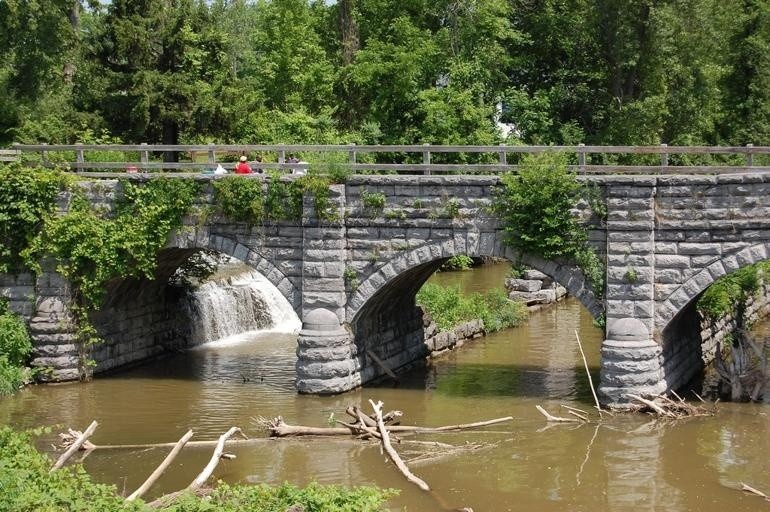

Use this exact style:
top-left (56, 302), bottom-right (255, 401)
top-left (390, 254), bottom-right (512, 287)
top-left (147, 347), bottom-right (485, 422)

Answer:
top-left (287, 153), bottom-right (300, 172)
top-left (235, 155), bottom-right (252, 176)
top-left (252, 152), bottom-right (263, 175)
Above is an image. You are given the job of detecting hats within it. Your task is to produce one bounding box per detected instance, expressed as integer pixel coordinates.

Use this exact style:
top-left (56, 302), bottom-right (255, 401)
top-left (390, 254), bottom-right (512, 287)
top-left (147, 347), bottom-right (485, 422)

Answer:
top-left (240, 156), bottom-right (247, 162)
